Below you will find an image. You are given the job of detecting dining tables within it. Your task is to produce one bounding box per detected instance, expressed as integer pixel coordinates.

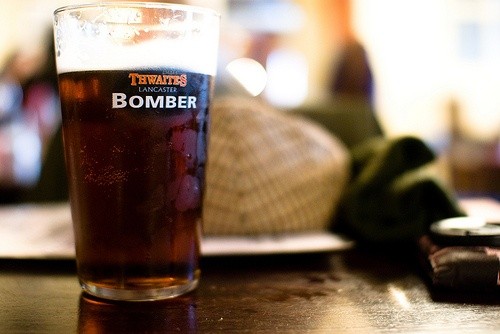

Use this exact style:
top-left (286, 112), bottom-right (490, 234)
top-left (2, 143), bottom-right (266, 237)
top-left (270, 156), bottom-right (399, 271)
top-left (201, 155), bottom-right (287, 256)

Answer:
top-left (0, 232), bottom-right (500, 334)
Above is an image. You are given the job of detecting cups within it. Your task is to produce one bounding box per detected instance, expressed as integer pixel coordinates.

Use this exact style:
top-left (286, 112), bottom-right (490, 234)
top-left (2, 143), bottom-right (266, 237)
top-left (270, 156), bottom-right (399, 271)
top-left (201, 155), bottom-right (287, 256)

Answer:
top-left (52, 3), bottom-right (221, 302)
